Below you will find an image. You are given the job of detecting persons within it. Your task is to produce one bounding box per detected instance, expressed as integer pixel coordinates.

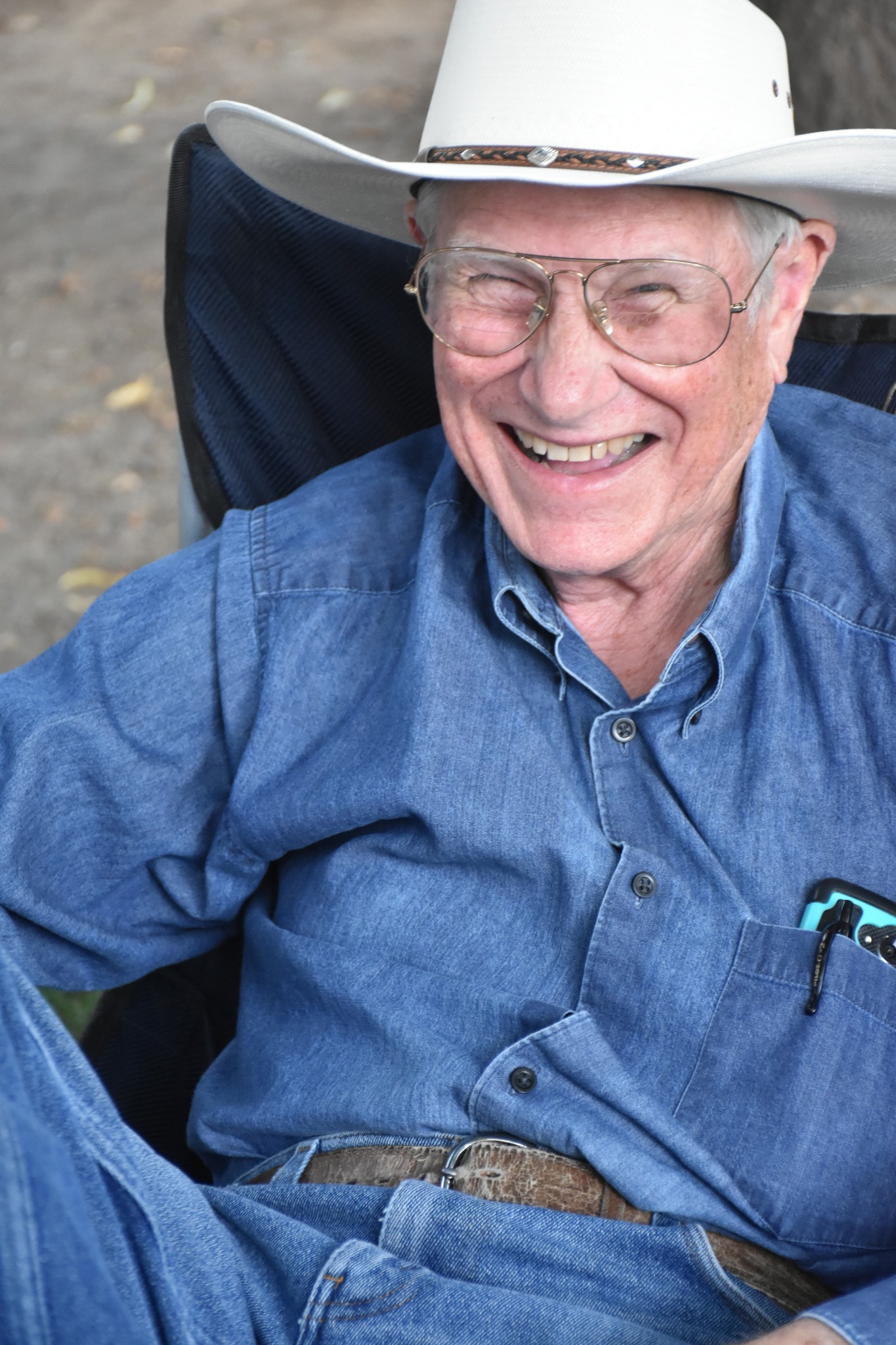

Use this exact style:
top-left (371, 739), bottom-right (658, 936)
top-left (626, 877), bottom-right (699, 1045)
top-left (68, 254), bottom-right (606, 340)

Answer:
top-left (0, 0), bottom-right (896, 1345)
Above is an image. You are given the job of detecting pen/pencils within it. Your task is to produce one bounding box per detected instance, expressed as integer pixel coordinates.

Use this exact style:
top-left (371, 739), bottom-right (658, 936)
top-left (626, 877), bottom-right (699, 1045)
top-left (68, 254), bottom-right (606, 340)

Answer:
top-left (805, 902), bottom-right (854, 1016)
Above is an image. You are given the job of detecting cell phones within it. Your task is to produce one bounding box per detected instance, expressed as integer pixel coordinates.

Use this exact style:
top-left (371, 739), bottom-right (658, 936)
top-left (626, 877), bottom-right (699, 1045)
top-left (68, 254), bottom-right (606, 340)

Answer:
top-left (798, 876), bottom-right (896, 967)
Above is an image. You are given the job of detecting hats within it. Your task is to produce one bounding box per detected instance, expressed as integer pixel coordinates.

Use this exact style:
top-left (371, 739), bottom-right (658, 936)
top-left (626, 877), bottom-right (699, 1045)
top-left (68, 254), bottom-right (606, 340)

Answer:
top-left (204, 0), bottom-right (896, 316)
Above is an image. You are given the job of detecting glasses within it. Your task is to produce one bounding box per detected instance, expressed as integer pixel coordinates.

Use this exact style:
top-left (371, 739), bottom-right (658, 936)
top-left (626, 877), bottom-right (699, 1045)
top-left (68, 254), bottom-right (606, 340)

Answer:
top-left (404, 229), bottom-right (789, 367)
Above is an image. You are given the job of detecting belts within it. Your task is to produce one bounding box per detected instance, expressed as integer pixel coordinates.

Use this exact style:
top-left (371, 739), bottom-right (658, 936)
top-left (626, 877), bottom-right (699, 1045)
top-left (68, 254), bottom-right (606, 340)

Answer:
top-left (241, 1134), bottom-right (842, 1319)
